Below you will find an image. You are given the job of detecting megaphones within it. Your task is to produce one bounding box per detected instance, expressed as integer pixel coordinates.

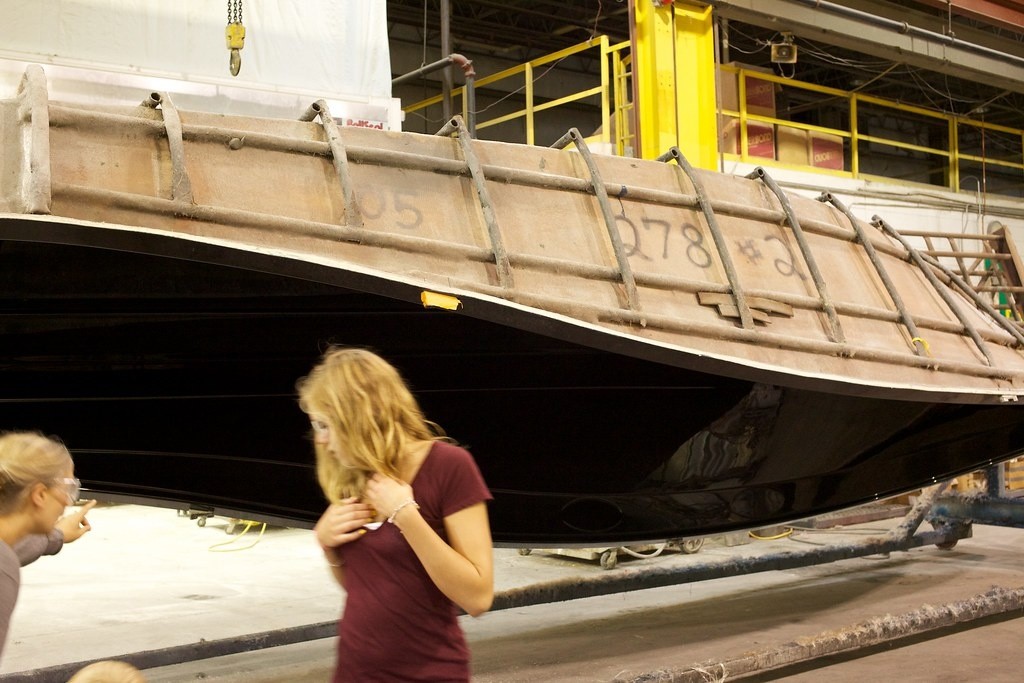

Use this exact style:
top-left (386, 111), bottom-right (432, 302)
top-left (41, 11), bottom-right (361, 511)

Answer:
top-left (771, 44), bottom-right (797, 63)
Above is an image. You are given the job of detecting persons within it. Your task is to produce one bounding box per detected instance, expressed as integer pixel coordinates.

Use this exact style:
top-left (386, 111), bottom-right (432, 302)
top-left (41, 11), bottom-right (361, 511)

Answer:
top-left (296, 350), bottom-right (495, 683)
top-left (0, 432), bottom-right (145, 683)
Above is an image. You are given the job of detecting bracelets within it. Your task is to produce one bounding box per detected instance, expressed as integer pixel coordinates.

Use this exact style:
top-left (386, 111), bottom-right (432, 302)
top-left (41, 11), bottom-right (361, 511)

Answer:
top-left (327, 559), bottom-right (346, 567)
top-left (388, 500), bottom-right (420, 523)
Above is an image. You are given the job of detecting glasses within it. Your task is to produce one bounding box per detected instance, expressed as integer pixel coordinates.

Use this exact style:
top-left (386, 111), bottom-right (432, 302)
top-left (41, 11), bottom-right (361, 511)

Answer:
top-left (311, 420), bottom-right (331, 433)
top-left (20, 478), bottom-right (81, 505)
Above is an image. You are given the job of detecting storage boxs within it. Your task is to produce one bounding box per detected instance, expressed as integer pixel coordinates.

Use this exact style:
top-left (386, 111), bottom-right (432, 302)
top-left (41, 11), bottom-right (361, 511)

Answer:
top-left (566, 100), bottom-right (633, 156)
top-left (716, 60), bottom-right (844, 169)
